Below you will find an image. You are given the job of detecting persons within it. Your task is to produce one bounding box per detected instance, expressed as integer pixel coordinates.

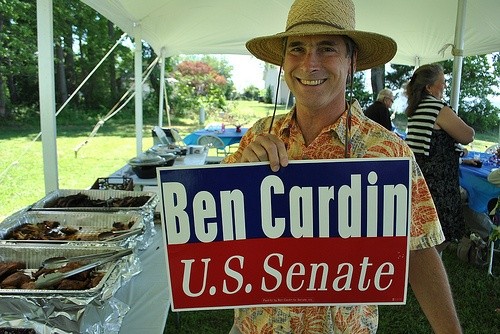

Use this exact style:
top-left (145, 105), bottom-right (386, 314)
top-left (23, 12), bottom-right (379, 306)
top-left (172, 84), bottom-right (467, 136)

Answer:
top-left (364, 88), bottom-right (395, 131)
top-left (221, 0), bottom-right (462, 334)
top-left (406, 63), bottom-right (475, 259)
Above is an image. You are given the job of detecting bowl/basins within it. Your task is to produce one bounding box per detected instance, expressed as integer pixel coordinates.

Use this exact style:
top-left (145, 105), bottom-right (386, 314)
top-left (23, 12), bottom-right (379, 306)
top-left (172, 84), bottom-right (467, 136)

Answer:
top-left (128, 154), bottom-right (175, 179)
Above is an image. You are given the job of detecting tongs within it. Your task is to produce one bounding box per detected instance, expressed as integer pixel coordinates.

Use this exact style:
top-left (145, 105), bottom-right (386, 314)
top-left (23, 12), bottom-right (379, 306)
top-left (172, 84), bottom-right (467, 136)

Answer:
top-left (98, 228), bottom-right (145, 242)
top-left (34, 248), bottom-right (135, 289)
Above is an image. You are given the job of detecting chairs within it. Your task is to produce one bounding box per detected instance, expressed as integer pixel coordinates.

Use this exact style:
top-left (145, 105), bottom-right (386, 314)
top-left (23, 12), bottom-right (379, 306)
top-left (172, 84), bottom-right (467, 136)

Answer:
top-left (197, 135), bottom-right (227, 164)
top-left (482, 200), bottom-right (500, 279)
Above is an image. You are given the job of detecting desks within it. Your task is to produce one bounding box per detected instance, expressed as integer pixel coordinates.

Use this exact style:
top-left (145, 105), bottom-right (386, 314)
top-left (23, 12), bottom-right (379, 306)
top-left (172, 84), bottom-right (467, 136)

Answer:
top-left (106, 145), bottom-right (209, 190)
top-left (183, 128), bottom-right (249, 154)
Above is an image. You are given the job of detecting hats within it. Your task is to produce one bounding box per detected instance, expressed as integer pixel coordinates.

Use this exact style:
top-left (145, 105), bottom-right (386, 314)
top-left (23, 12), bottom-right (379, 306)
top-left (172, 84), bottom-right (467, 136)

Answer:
top-left (246, 0), bottom-right (397, 71)
